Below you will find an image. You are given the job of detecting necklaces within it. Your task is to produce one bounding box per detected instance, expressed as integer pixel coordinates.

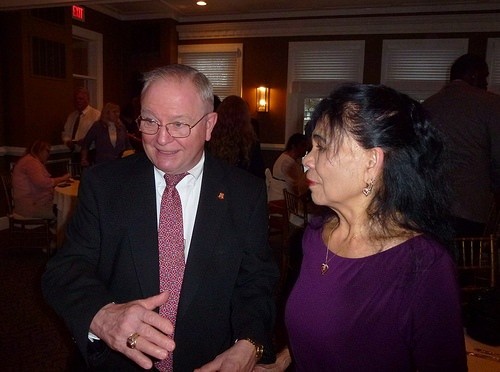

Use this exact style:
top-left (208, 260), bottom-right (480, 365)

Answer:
top-left (322, 222), bottom-right (370, 275)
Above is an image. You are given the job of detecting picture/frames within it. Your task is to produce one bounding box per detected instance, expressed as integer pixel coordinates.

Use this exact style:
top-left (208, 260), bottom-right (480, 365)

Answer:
top-left (29, 7), bottom-right (65, 26)
top-left (29, 36), bottom-right (67, 81)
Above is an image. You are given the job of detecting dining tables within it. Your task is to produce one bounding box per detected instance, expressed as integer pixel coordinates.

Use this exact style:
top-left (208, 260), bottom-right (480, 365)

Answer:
top-left (53, 179), bottom-right (80, 252)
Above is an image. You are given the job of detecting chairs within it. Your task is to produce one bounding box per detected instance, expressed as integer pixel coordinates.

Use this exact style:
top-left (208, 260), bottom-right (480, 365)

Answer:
top-left (279, 188), bottom-right (314, 286)
top-left (2, 172), bottom-right (50, 262)
top-left (68, 163), bottom-right (94, 181)
top-left (443, 233), bottom-right (500, 340)
top-left (265, 168), bottom-right (291, 244)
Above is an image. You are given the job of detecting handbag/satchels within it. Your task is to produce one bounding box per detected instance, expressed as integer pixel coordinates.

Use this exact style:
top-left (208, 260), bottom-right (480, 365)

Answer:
top-left (466, 287), bottom-right (500, 346)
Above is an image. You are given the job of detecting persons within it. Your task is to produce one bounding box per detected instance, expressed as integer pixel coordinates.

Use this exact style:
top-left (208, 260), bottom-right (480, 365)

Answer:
top-left (81, 102), bottom-right (128, 166)
top-left (40, 62), bottom-right (281, 372)
top-left (10, 140), bottom-right (72, 239)
top-left (62, 85), bottom-right (102, 180)
top-left (251, 82), bottom-right (469, 372)
top-left (213, 96), bottom-right (315, 227)
top-left (416, 53), bottom-right (500, 266)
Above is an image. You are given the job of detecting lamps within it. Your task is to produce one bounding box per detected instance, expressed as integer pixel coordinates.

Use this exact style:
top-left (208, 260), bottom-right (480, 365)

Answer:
top-left (256, 83), bottom-right (270, 112)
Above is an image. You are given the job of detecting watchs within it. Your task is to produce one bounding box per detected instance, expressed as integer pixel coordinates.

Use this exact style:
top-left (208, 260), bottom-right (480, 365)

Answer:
top-left (234, 337), bottom-right (264, 362)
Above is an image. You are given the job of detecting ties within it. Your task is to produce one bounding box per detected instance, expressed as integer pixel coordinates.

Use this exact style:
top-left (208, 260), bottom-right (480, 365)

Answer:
top-left (71, 112), bottom-right (83, 140)
top-left (154, 172), bottom-right (186, 372)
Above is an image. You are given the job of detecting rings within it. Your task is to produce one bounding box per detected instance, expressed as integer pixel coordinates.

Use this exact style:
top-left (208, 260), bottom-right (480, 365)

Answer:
top-left (126, 333), bottom-right (140, 349)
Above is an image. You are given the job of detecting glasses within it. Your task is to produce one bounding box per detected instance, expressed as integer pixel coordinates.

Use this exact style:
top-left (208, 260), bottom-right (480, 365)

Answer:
top-left (135, 112), bottom-right (210, 138)
